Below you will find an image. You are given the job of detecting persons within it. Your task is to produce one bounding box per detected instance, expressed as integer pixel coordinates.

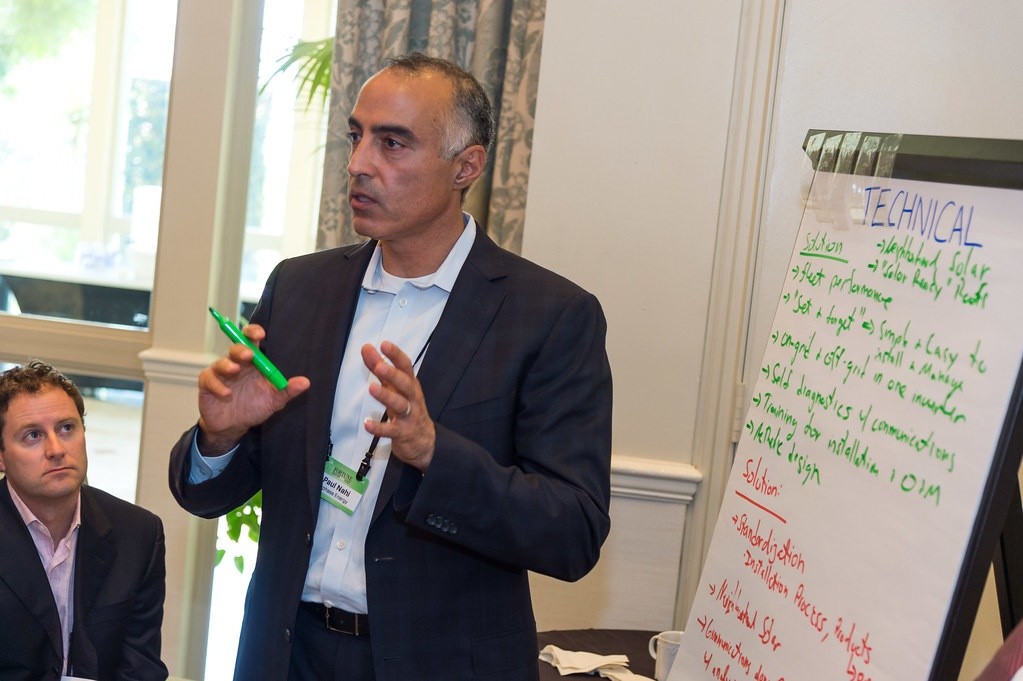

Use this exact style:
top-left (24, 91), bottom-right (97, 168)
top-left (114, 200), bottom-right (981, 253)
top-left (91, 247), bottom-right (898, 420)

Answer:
top-left (0, 360), bottom-right (170, 681)
top-left (168, 51), bottom-right (614, 681)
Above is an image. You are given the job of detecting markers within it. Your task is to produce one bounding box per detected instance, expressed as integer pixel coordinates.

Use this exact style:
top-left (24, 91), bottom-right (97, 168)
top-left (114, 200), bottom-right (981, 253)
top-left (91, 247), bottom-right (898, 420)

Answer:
top-left (204, 306), bottom-right (288, 391)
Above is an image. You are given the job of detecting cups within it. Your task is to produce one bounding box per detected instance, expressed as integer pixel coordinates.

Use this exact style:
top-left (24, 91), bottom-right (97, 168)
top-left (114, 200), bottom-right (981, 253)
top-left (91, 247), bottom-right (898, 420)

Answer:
top-left (649, 631), bottom-right (685, 681)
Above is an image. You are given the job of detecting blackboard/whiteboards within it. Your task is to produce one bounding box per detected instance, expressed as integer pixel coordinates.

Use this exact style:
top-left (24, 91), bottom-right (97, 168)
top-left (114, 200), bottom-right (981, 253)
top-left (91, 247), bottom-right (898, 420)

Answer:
top-left (662, 127), bottom-right (1023, 681)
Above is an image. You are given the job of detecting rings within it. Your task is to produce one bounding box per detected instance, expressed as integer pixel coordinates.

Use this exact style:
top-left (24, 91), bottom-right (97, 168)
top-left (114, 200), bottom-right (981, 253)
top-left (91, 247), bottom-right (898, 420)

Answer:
top-left (395, 402), bottom-right (410, 418)
